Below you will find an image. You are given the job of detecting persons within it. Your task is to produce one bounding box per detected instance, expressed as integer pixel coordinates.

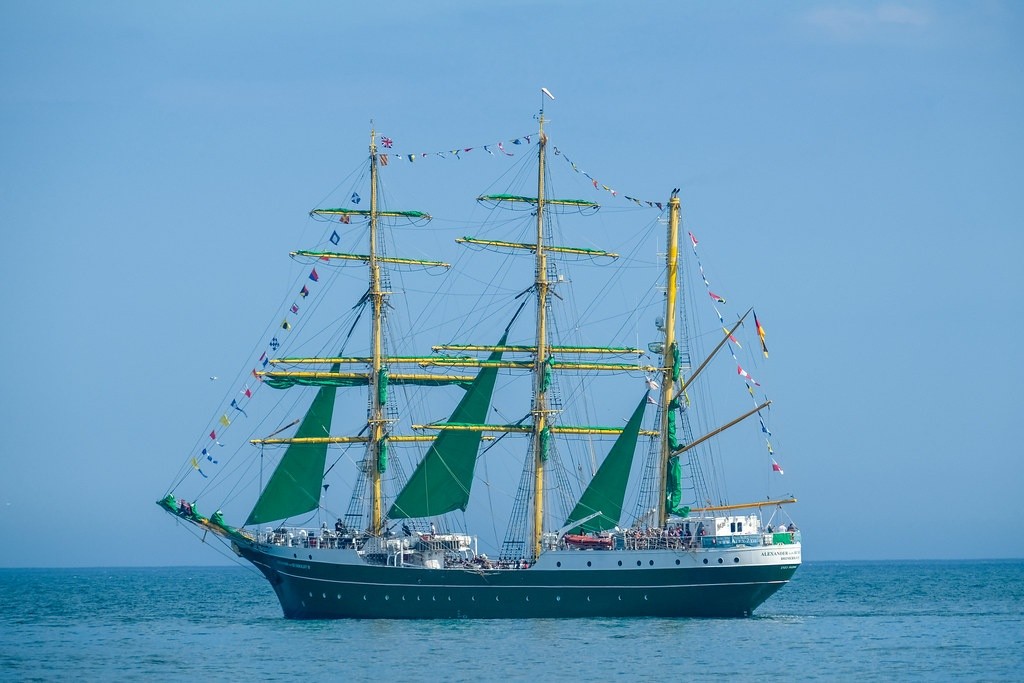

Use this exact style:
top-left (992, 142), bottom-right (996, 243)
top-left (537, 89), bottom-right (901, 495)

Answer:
top-left (563, 521), bottom-right (706, 549)
top-left (787, 524), bottom-right (798, 539)
top-left (767, 525), bottom-right (775, 533)
top-left (430, 522), bottom-right (435, 537)
top-left (777, 523), bottom-right (787, 532)
top-left (384, 528), bottom-right (391, 537)
top-left (402, 523), bottom-right (412, 536)
top-left (321, 522), bottom-right (329, 536)
top-left (335, 518), bottom-right (346, 531)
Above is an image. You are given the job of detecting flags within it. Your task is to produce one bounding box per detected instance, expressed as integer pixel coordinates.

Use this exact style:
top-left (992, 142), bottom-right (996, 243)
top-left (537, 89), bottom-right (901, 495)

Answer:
top-left (308, 191), bottom-right (361, 281)
top-left (191, 285), bottom-right (309, 479)
top-left (534, 108), bottom-right (786, 476)
top-left (378, 135), bottom-right (531, 167)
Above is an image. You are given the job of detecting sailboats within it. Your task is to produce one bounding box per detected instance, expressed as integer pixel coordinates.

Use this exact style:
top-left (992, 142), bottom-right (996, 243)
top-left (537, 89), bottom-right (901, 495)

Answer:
top-left (156, 88), bottom-right (802, 620)
